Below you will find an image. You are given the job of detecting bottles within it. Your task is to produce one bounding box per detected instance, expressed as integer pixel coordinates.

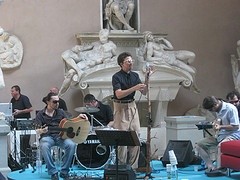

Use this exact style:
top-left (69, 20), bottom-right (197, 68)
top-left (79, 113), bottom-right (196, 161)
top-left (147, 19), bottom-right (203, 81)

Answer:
top-left (36, 158), bottom-right (42, 173)
top-left (201, 159), bottom-right (206, 169)
top-left (171, 165), bottom-right (178, 180)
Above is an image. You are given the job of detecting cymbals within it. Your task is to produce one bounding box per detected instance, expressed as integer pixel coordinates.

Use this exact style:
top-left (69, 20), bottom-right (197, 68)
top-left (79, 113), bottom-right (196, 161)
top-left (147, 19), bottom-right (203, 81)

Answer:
top-left (74, 107), bottom-right (100, 112)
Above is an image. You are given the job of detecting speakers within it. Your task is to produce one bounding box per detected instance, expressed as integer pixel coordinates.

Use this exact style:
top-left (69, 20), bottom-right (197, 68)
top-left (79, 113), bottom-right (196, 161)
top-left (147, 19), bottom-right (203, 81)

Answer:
top-left (104, 165), bottom-right (137, 180)
top-left (162, 140), bottom-right (194, 167)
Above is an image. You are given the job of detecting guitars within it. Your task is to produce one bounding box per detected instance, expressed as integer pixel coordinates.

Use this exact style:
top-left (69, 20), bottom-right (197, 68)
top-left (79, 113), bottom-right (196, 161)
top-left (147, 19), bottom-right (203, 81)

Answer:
top-left (212, 119), bottom-right (222, 132)
top-left (33, 118), bottom-right (90, 143)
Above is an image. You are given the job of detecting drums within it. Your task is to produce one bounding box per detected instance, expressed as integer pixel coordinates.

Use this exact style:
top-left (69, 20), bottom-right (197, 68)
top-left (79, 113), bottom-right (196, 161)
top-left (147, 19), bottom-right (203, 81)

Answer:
top-left (92, 127), bottom-right (113, 143)
top-left (75, 133), bottom-right (111, 169)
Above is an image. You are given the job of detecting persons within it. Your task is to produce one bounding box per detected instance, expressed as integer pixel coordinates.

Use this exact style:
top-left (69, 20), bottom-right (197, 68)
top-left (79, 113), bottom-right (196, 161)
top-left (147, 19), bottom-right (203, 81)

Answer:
top-left (112, 51), bottom-right (157, 176)
top-left (57, 28), bottom-right (119, 98)
top-left (34, 92), bottom-right (88, 180)
top-left (194, 90), bottom-right (240, 177)
top-left (82, 94), bottom-right (115, 127)
top-left (103, 0), bottom-right (136, 31)
top-left (142, 31), bottom-right (201, 95)
top-left (10, 85), bottom-right (34, 169)
top-left (50, 87), bottom-right (74, 120)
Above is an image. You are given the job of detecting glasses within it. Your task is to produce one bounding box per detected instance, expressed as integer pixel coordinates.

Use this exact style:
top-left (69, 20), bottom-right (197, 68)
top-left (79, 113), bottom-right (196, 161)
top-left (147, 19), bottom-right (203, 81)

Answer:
top-left (48, 100), bottom-right (60, 104)
top-left (229, 99), bottom-right (240, 104)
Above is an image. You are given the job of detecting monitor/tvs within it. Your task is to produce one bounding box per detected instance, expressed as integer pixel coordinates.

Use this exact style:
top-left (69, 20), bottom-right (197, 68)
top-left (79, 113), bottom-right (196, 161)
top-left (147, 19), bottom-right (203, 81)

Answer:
top-left (0, 103), bottom-right (12, 116)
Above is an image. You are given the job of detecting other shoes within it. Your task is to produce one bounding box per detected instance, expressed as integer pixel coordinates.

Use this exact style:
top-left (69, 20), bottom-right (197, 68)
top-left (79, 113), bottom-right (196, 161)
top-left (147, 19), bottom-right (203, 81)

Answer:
top-left (51, 172), bottom-right (59, 180)
top-left (134, 171), bottom-right (146, 178)
top-left (60, 173), bottom-right (73, 180)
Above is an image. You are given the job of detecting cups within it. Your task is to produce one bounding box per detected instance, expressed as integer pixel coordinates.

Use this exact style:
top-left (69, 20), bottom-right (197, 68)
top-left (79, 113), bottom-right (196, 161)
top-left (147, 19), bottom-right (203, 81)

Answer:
top-left (168, 150), bottom-right (178, 165)
top-left (194, 166), bottom-right (198, 172)
top-left (166, 164), bottom-right (171, 179)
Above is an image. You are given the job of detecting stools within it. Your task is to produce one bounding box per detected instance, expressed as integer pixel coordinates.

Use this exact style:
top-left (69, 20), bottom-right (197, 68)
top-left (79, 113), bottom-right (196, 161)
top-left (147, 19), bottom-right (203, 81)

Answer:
top-left (220, 140), bottom-right (240, 177)
top-left (36, 143), bottom-right (60, 178)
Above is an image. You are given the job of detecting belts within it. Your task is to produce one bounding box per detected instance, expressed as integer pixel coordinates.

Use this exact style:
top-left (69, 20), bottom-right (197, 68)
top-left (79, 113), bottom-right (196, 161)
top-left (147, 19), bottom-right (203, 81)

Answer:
top-left (114, 99), bottom-right (135, 103)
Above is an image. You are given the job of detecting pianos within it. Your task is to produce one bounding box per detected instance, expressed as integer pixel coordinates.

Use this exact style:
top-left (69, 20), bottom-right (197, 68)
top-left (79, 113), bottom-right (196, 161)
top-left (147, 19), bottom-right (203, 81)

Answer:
top-left (194, 122), bottom-right (217, 171)
